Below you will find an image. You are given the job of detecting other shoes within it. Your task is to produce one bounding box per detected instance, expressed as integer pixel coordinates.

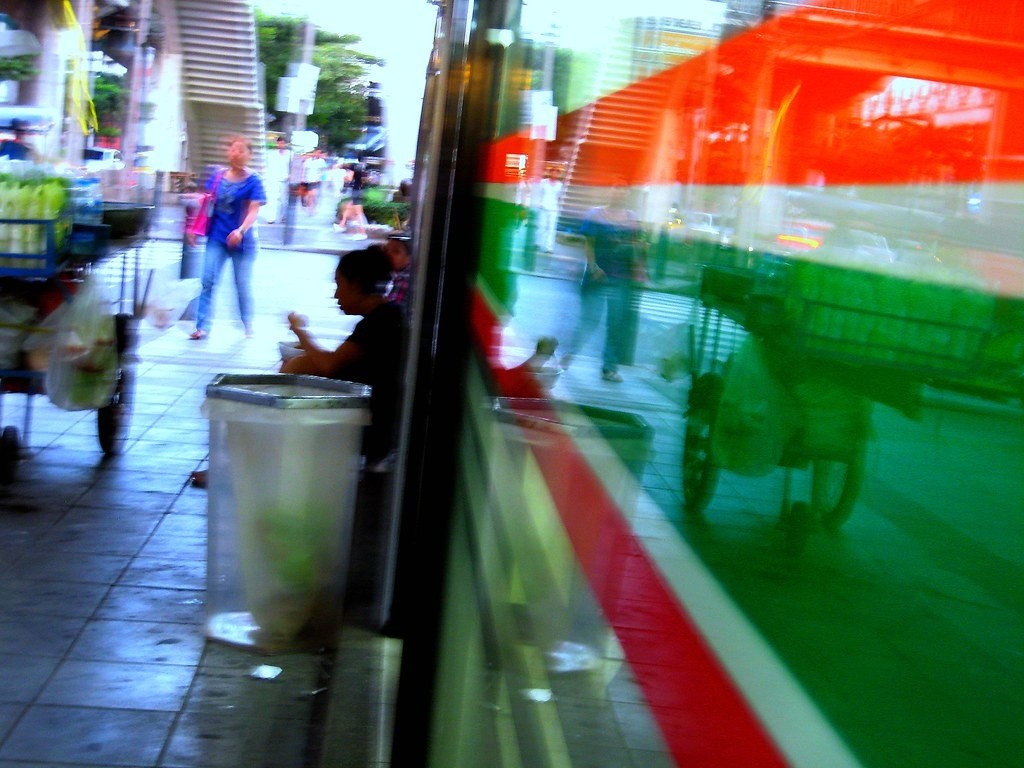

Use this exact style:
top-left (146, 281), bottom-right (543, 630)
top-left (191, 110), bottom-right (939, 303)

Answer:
top-left (335, 227), bottom-right (347, 233)
top-left (190, 330), bottom-right (209, 338)
top-left (351, 234), bottom-right (367, 240)
top-left (604, 372), bottom-right (623, 381)
top-left (245, 330), bottom-right (254, 338)
top-left (192, 470), bottom-right (208, 489)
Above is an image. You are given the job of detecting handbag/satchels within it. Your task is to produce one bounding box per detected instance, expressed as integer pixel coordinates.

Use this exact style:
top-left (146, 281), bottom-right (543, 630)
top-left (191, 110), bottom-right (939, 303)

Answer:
top-left (45, 276), bottom-right (118, 410)
top-left (190, 168), bottom-right (224, 237)
top-left (709, 331), bottom-right (786, 476)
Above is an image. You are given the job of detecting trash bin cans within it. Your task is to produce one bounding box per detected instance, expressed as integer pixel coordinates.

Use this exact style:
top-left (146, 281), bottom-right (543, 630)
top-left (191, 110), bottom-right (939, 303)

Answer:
top-left (201, 372), bottom-right (384, 655)
top-left (486, 389), bottom-right (656, 683)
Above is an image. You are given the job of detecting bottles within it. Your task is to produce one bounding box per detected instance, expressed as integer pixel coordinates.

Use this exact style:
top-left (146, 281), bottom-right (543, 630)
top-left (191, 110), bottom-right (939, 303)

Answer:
top-left (72, 179), bottom-right (103, 243)
top-left (753, 251), bottom-right (786, 296)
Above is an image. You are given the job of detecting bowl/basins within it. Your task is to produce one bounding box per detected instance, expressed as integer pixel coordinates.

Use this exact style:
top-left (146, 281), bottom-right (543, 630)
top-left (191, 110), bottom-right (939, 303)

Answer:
top-left (526, 367), bottom-right (563, 389)
top-left (704, 265), bottom-right (780, 303)
top-left (279, 341), bottom-right (306, 361)
top-left (102, 202), bottom-right (155, 236)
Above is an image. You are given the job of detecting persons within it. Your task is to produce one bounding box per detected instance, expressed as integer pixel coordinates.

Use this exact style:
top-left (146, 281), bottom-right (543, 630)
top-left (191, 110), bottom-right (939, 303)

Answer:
top-left (188, 133), bottom-right (266, 340)
top-left (0, 119), bottom-right (408, 485)
top-left (559, 179), bottom-right (635, 383)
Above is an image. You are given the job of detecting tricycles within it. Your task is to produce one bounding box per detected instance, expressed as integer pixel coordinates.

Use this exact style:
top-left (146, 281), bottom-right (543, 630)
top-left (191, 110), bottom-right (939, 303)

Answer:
top-left (678, 264), bottom-right (996, 530)
top-left (0, 202), bottom-right (162, 503)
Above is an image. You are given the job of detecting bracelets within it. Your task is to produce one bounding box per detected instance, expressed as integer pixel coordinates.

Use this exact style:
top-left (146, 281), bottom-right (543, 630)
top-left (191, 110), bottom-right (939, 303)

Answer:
top-left (237, 226), bottom-right (245, 234)
